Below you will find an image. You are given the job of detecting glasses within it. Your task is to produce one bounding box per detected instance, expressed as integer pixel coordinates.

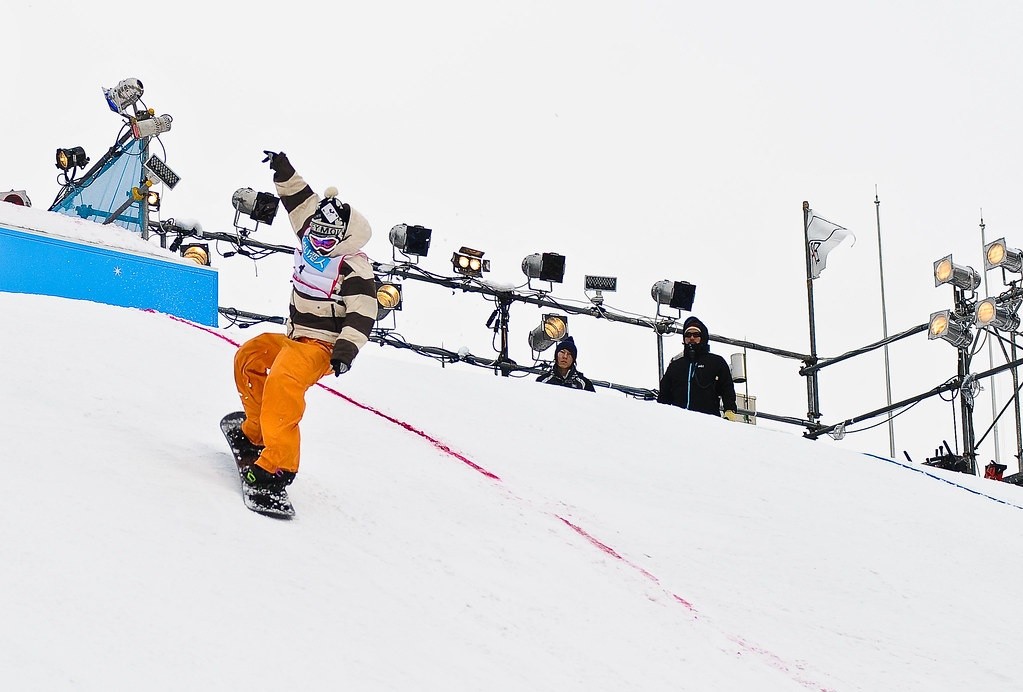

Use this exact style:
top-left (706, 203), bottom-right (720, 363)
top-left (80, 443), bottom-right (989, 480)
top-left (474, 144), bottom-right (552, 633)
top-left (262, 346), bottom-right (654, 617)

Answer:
top-left (684, 332), bottom-right (701, 338)
top-left (309, 234), bottom-right (339, 252)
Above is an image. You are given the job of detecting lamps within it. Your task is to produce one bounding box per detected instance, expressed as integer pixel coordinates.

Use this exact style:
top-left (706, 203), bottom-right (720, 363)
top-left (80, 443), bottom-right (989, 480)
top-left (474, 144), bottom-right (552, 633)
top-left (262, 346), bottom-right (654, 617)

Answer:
top-left (527, 313), bottom-right (568, 363)
top-left (928, 310), bottom-right (973, 349)
top-left (974, 296), bottom-right (1022, 332)
top-left (0, 189), bottom-right (32, 207)
top-left (933, 253), bottom-right (981, 298)
top-left (373, 281), bottom-right (402, 321)
top-left (101, 77), bottom-right (173, 141)
top-left (584, 275), bottom-right (617, 304)
top-left (451, 246), bottom-right (485, 279)
top-left (147, 191), bottom-right (160, 212)
top-left (143, 153), bottom-right (182, 192)
top-left (982, 237), bottom-right (1023, 286)
top-left (55, 146), bottom-right (90, 186)
top-left (178, 242), bottom-right (211, 266)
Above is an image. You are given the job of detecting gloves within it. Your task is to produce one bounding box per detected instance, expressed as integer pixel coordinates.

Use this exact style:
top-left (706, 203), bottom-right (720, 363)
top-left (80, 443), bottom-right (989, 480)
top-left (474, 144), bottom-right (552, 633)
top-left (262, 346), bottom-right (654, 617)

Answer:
top-left (262, 150), bottom-right (278, 170)
top-left (724, 410), bottom-right (736, 422)
top-left (330, 360), bottom-right (350, 378)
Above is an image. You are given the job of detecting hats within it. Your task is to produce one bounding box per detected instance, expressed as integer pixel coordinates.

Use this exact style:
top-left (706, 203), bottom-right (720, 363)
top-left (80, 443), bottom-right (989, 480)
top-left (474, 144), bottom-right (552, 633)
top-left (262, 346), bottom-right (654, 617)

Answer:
top-left (554, 336), bottom-right (577, 360)
top-left (684, 320), bottom-right (703, 335)
top-left (309, 186), bottom-right (348, 236)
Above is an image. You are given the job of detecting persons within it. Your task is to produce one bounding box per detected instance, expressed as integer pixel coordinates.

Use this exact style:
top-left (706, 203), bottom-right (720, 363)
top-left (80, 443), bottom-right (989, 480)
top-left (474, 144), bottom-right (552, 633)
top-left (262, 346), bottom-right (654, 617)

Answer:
top-left (234, 150), bottom-right (378, 491)
top-left (657, 316), bottom-right (738, 422)
top-left (535, 336), bottom-right (596, 393)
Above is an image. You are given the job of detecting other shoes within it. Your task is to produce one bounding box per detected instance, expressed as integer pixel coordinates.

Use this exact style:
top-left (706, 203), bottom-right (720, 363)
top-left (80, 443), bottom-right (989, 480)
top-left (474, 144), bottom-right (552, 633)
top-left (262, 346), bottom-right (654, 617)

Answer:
top-left (232, 430), bottom-right (266, 450)
top-left (242, 464), bottom-right (295, 486)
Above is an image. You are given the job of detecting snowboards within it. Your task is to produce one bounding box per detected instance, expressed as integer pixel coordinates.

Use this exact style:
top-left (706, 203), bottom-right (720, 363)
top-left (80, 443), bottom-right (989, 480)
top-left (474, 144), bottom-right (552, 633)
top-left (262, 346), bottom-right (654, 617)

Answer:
top-left (220, 410), bottom-right (298, 518)
